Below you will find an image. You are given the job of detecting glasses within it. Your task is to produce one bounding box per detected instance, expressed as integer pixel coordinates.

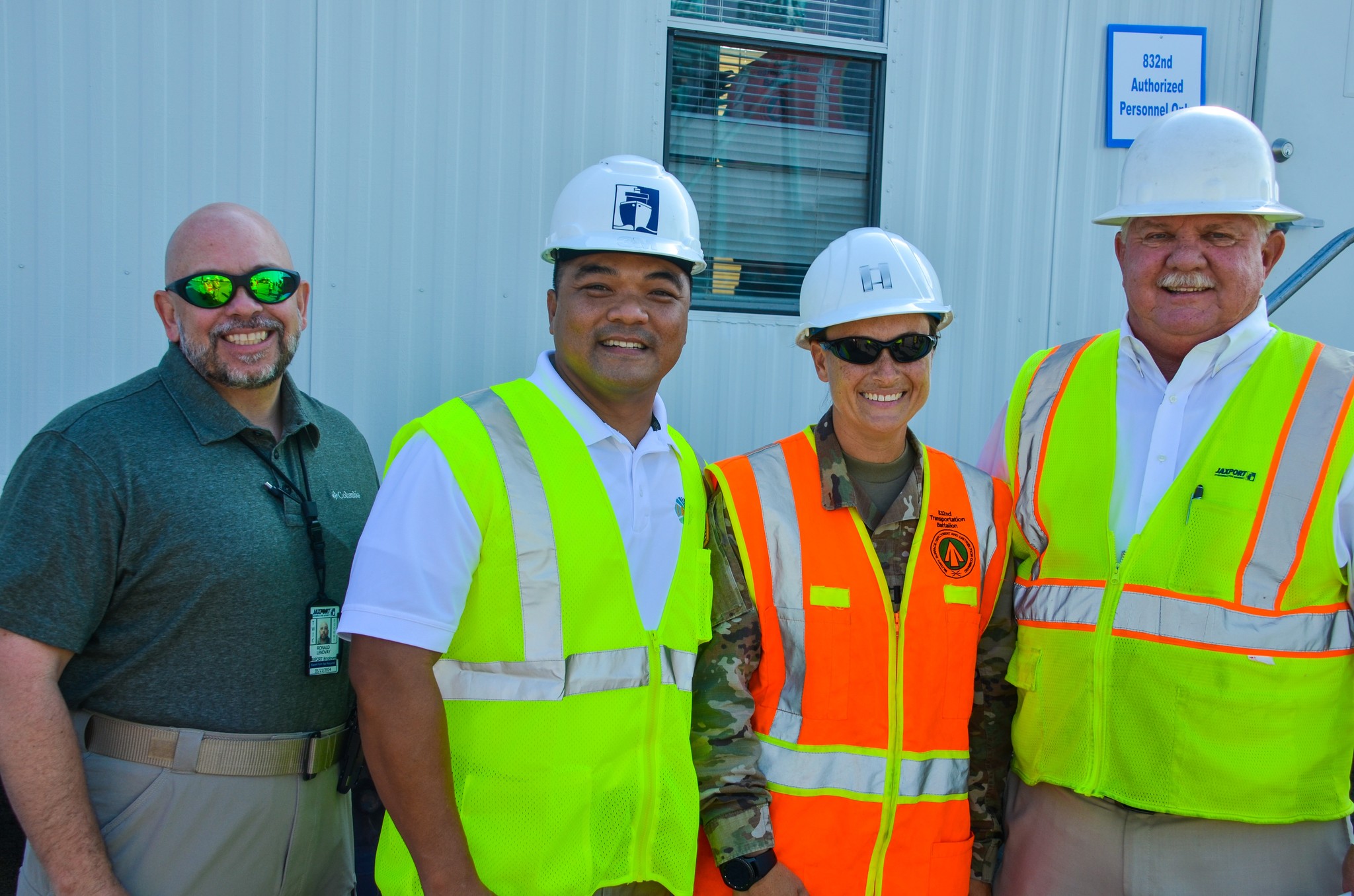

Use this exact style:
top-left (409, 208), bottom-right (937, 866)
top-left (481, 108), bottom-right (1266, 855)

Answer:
top-left (821, 333), bottom-right (937, 365)
top-left (167, 268), bottom-right (300, 309)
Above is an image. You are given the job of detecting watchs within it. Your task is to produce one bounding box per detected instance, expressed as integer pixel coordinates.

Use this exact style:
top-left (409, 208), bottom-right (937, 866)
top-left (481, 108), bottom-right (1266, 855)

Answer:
top-left (719, 848), bottom-right (777, 891)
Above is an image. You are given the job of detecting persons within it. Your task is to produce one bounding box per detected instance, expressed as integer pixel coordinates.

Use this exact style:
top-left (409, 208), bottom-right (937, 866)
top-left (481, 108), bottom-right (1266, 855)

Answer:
top-left (336, 152), bottom-right (712, 896)
top-left (0, 202), bottom-right (381, 896)
top-left (977, 105), bottom-right (1354, 896)
top-left (689, 227), bottom-right (1017, 896)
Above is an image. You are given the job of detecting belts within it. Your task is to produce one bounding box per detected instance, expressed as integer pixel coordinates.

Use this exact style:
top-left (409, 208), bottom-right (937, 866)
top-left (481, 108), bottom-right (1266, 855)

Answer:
top-left (85, 717), bottom-right (342, 780)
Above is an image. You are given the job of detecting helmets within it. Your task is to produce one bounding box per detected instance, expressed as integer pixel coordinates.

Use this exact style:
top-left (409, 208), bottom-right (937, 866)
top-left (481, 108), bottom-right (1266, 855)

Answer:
top-left (1090, 105), bottom-right (1305, 227)
top-left (541, 154), bottom-right (708, 275)
top-left (794, 226), bottom-right (954, 351)
